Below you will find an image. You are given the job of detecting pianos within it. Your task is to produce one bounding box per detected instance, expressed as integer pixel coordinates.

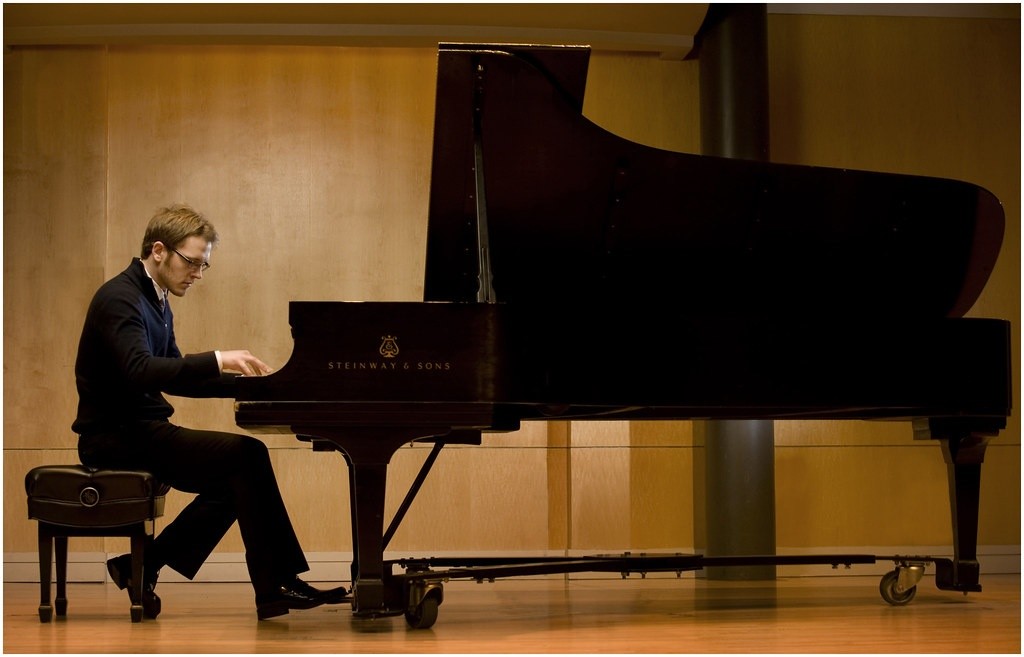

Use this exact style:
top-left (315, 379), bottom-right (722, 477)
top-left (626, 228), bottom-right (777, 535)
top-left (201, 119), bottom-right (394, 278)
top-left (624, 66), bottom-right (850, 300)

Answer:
top-left (234, 40), bottom-right (1013, 629)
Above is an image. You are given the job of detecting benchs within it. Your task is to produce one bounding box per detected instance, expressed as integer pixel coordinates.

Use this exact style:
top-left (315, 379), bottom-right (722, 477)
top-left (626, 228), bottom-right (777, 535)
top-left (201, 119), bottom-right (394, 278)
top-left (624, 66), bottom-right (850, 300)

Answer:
top-left (25, 465), bottom-right (170, 622)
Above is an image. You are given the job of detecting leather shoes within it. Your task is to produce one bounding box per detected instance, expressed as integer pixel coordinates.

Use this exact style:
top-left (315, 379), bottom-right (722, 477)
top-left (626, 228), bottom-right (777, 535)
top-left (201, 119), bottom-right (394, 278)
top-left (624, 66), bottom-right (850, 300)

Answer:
top-left (255, 575), bottom-right (347, 620)
top-left (107, 554), bottom-right (161, 620)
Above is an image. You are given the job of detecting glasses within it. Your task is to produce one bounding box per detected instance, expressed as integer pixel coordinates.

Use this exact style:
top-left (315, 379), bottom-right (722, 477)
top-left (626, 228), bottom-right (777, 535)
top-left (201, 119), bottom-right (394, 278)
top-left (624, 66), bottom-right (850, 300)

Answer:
top-left (169, 246), bottom-right (210, 272)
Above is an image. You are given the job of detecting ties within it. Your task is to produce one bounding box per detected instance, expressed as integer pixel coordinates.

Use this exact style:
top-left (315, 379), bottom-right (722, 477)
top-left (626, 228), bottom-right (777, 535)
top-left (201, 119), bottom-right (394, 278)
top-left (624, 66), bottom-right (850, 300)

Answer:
top-left (159, 296), bottom-right (166, 314)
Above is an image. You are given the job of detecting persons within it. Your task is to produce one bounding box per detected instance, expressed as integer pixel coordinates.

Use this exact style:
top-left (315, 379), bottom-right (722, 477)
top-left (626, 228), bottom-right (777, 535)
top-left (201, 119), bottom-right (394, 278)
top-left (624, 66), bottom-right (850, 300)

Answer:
top-left (71, 202), bottom-right (348, 621)
